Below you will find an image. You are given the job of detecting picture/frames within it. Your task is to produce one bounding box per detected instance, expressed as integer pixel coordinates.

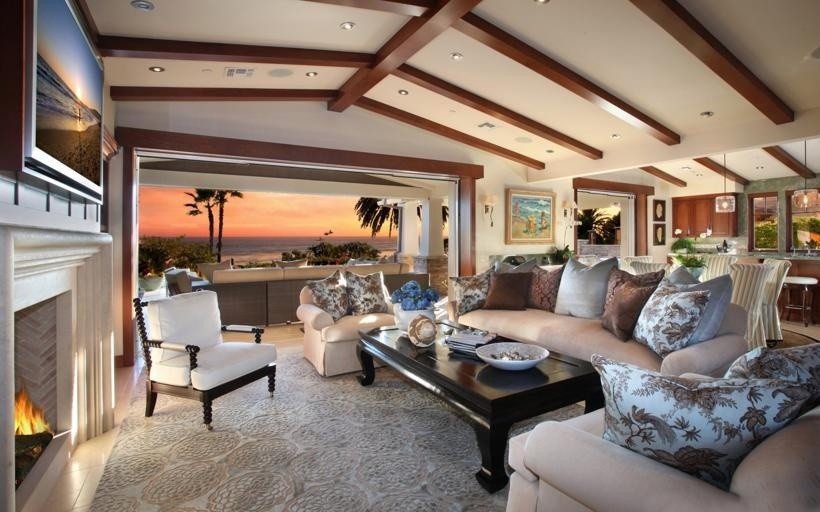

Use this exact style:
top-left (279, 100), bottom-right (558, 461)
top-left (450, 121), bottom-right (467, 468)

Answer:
top-left (652, 199), bottom-right (667, 221)
top-left (652, 223), bottom-right (666, 246)
top-left (504, 188), bottom-right (557, 245)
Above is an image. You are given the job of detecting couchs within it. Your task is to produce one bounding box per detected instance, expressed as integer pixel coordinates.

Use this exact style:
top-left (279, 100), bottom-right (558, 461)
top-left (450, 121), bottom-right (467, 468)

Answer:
top-left (506, 373), bottom-right (820, 511)
top-left (457, 302), bottom-right (749, 376)
top-left (134, 289), bottom-right (277, 432)
top-left (296, 284), bottom-right (396, 377)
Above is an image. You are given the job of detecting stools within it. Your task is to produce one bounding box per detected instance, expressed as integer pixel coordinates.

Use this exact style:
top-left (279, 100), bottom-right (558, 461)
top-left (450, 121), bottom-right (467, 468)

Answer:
top-left (777, 275), bottom-right (818, 327)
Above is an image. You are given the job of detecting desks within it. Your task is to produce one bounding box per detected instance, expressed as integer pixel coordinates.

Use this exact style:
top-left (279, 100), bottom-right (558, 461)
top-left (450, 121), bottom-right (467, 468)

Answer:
top-left (759, 256), bottom-right (820, 325)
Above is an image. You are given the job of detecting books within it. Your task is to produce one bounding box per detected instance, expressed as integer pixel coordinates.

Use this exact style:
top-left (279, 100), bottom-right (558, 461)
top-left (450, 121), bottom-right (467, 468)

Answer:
top-left (446, 328), bottom-right (498, 362)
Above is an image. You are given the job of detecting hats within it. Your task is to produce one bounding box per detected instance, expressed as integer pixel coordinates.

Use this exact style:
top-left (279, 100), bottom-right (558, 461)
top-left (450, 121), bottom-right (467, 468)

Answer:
top-left (528, 215), bottom-right (536, 221)
top-left (408, 314), bottom-right (437, 347)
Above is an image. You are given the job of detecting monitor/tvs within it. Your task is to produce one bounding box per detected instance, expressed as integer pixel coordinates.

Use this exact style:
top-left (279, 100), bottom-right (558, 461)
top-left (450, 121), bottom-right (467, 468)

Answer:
top-left (25, 0), bottom-right (105, 195)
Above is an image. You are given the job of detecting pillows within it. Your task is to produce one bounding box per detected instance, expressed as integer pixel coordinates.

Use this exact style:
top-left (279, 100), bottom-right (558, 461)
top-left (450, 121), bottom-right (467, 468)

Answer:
top-left (448, 273), bottom-right (490, 317)
top-left (343, 270), bottom-right (388, 317)
top-left (599, 280), bottom-right (656, 343)
top-left (304, 268), bottom-right (349, 322)
top-left (606, 265), bottom-right (665, 288)
top-left (724, 343), bottom-right (820, 397)
top-left (526, 264), bottom-right (565, 313)
top-left (482, 270), bottom-right (533, 310)
top-left (668, 265), bottom-right (733, 345)
top-left (589, 355), bottom-right (817, 487)
top-left (555, 257), bottom-right (618, 319)
top-left (632, 278), bottom-right (712, 357)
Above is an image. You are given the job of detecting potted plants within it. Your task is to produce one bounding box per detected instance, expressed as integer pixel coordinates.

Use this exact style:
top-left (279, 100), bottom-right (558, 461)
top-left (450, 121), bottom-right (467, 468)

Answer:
top-left (390, 281), bottom-right (439, 330)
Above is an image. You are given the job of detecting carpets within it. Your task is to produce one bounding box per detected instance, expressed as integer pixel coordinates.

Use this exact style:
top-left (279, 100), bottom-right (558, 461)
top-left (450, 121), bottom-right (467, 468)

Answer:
top-left (90, 343), bottom-right (587, 512)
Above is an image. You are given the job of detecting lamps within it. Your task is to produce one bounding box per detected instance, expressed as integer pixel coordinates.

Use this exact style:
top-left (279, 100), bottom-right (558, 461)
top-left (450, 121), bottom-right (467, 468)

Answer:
top-left (792, 141), bottom-right (820, 213)
top-left (484, 192), bottom-right (500, 227)
top-left (560, 197), bottom-right (577, 230)
top-left (716, 154), bottom-right (736, 213)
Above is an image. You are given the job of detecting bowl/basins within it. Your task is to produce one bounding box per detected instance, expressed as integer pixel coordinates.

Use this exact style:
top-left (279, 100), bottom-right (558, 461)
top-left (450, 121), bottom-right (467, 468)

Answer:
top-left (475, 342), bottom-right (550, 370)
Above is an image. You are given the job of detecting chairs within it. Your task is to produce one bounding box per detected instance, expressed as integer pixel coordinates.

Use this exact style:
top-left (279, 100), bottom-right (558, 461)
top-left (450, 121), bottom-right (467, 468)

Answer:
top-left (630, 261), bottom-right (666, 274)
top-left (700, 254), bottom-right (737, 281)
top-left (730, 264), bottom-right (776, 350)
top-left (763, 258), bottom-right (792, 340)
top-left (624, 256), bottom-right (653, 272)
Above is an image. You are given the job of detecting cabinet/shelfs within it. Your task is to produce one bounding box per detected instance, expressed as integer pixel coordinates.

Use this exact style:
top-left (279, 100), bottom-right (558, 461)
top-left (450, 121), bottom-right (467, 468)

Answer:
top-left (672, 192), bottom-right (738, 238)
